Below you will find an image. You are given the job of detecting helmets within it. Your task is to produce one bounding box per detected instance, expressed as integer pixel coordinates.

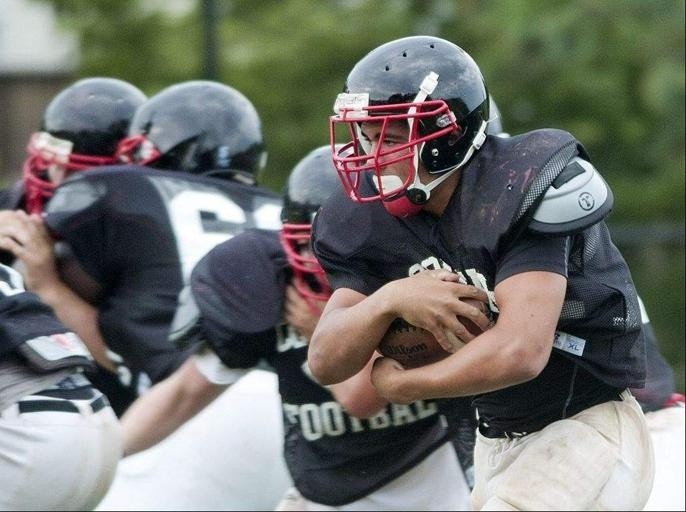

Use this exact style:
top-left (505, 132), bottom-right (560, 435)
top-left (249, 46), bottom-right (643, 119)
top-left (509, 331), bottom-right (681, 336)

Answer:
top-left (329, 34), bottom-right (490, 216)
top-left (281, 144), bottom-right (356, 301)
top-left (23, 76), bottom-right (148, 212)
top-left (117, 79), bottom-right (263, 178)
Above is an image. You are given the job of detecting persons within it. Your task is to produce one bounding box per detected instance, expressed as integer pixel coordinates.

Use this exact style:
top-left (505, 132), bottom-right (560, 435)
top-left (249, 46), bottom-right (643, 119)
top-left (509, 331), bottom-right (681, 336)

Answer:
top-left (118, 143), bottom-right (476, 510)
top-left (629, 293), bottom-right (685, 510)
top-left (20, 81), bottom-right (285, 510)
top-left (308, 36), bottom-right (654, 510)
top-left (0, 77), bottom-right (149, 415)
top-left (0, 262), bottom-right (120, 510)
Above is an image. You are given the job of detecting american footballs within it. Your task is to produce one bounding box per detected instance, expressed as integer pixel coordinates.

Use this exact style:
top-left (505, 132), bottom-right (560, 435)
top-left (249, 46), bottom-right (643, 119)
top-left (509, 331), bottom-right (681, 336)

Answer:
top-left (376, 296), bottom-right (489, 368)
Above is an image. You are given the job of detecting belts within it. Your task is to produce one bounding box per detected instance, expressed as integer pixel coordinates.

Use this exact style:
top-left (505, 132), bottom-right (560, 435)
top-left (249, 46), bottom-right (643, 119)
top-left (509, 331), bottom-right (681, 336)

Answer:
top-left (20, 395), bottom-right (105, 413)
